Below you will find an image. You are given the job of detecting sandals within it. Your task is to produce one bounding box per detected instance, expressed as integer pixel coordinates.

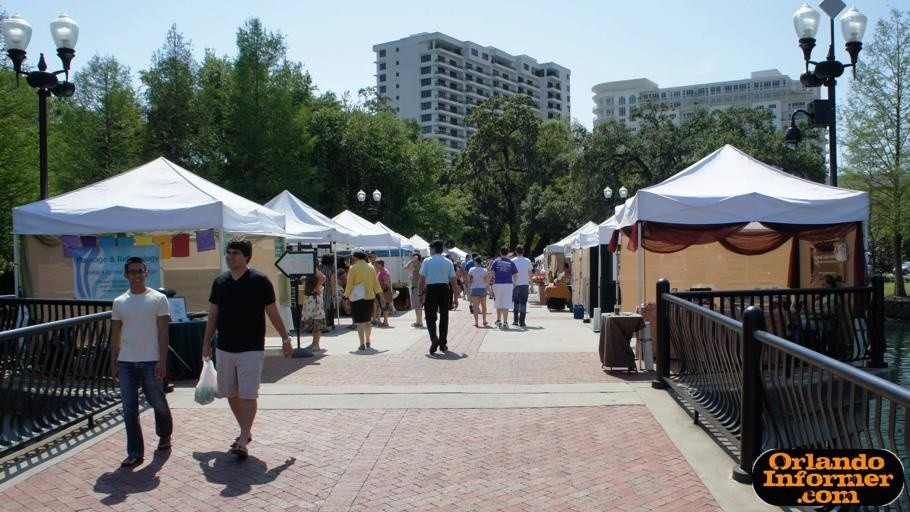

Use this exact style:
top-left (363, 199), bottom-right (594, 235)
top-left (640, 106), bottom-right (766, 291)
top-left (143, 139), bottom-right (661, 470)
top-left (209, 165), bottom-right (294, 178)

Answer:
top-left (228, 430), bottom-right (256, 458)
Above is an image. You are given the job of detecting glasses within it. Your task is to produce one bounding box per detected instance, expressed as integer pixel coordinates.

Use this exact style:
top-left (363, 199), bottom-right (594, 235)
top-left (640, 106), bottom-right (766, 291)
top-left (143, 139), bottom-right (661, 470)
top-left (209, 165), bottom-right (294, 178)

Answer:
top-left (225, 249), bottom-right (243, 258)
top-left (127, 269), bottom-right (148, 275)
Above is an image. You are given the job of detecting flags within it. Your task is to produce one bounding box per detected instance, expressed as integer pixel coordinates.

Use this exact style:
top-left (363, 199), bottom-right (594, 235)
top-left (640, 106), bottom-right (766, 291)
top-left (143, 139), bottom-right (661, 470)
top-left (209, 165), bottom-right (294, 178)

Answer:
top-left (61, 227), bottom-right (218, 259)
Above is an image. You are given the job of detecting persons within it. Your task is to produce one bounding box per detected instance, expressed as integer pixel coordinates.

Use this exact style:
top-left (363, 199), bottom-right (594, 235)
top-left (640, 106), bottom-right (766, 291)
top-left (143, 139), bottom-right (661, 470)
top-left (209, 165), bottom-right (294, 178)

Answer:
top-left (336, 246), bottom-right (394, 353)
top-left (199, 234), bottom-right (294, 462)
top-left (416, 238), bottom-right (461, 354)
top-left (401, 252), bottom-right (425, 329)
top-left (813, 276), bottom-right (842, 314)
top-left (532, 263), bottom-right (540, 274)
top-left (299, 255), bottom-right (329, 353)
top-left (554, 262), bottom-right (571, 285)
top-left (447, 244), bottom-right (534, 329)
top-left (317, 253), bottom-right (339, 333)
top-left (107, 256), bottom-right (174, 470)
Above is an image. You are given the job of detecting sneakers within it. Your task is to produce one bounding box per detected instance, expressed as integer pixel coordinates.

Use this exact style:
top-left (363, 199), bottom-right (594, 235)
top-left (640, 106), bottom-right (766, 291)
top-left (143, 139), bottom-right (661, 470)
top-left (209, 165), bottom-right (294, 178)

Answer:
top-left (378, 322), bottom-right (390, 327)
top-left (410, 321), bottom-right (424, 328)
top-left (154, 434), bottom-right (174, 451)
top-left (357, 344), bottom-right (365, 350)
top-left (365, 342), bottom-right (371, 349)
top-left (304, 344), bottom-right (321, 352)
top-left (503, 321), bottom-right (510, 330)
top-left (119, 451), bottom-right (147, 469)
top-left (428, 336), bottom-right (440, 354)
top-left (439, 344), bottom-right (451, 352)
top-left (494, 319), bottom-right (502, 328)
top-left (511, 323), bottom-right (527, 328)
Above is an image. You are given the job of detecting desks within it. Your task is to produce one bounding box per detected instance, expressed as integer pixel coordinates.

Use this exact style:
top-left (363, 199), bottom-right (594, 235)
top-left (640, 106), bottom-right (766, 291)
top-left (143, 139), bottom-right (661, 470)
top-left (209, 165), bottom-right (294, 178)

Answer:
top-left (168, 309), bottom-right (221, 383)
top-left (596, 311), bottom-right (644, 376)
top-left (635, 301), bottom-right (820, 374)
top-left (392, 283), bottom-right (412, 311)
top-left (543, 282), bottom-right (570, 312)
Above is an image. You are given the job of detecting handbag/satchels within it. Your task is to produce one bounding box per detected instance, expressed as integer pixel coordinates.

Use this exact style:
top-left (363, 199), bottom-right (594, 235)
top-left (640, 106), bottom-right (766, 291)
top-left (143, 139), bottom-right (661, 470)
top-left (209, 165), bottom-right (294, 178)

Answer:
top-left (348, 282), bottom-right (366, 303)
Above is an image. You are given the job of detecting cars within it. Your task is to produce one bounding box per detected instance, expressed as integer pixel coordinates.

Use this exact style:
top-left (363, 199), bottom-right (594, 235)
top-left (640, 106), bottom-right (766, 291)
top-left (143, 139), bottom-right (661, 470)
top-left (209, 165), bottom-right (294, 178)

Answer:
top-left (892, 261), bottom-right (910, 275)
top-left (867, 251), bottom-right (872, 274)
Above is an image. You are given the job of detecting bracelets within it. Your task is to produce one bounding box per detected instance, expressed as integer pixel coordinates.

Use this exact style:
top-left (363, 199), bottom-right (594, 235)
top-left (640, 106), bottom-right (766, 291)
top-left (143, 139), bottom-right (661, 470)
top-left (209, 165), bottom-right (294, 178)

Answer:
top-left (418, 293), bottom-right (423, 297)
top-left (283, 337), bottom-right (292, 344)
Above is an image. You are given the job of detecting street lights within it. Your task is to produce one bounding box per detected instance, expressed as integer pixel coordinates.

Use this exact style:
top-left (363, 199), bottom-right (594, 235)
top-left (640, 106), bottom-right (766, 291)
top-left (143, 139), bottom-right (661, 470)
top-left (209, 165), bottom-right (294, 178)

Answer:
top-left (604, 185), bottom-right (628, 217)
top-left (356, 190), bottom-right (382, 222)
top-left (784, 5), bottom-right (869, 189)
top-left (3, 9), bottom-right (81, 200)
